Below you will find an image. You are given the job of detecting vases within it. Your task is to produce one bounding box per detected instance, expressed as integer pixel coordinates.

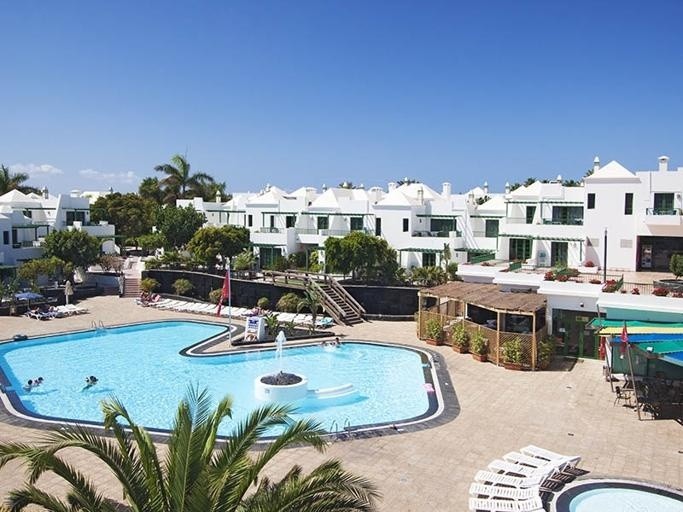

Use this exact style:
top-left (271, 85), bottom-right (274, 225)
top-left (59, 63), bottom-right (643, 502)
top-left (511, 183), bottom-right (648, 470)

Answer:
top-left (580, 265), bottom-right (598, 275)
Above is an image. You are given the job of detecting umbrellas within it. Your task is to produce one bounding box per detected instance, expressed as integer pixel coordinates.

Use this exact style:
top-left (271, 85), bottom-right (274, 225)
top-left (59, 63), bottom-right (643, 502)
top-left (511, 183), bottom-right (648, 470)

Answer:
top-left (16, 292), bottom-right (43, 310)
top-left (64, 280), bottom-right (73, 304)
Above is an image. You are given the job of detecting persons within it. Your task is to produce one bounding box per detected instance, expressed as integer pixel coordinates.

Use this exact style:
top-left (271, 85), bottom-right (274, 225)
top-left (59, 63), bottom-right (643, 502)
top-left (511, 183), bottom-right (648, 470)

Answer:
top-left (319, 341), bottom-right (328, 347)
top-left (79, 376), bottom-right (98, 391)
top-left (23, 379), bottom-right (32, 394)
top-left (333, 337), bottom-right (341, 346)
top-left (32, 377), bottom-right (44, 387)
top-left (30, 304), bottom-right (59, 314)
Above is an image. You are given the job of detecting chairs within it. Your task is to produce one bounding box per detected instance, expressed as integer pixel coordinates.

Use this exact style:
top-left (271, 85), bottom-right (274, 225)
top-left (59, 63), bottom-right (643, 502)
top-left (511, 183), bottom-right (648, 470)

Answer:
top-left (135, 293), bottom-right (333, 329)
top-left (613, 371), bottom-right (683, 421)
top-left (25, 304), bottom-right (87, 321)
top-left (468, 444), bottom-right (581, 512)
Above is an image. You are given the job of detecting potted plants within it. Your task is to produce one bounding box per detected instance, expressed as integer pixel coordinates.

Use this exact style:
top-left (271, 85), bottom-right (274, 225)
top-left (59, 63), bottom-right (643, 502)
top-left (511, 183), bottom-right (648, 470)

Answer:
top-left (451, 326), bottom-right (469, 353)
top-left (539, 335), bottom-right (554, 369)
top-left (501, 337), bottom-right (523, 371)
top-left (425, 319), bottom-right (443, 346)
top-left (468, 330), bottom-right (488, 361)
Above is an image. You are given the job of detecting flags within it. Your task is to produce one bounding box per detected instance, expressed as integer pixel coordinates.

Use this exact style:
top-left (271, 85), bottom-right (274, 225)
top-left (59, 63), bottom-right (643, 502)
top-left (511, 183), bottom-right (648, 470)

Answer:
top-left (216, 270), bottom-right (230, 316)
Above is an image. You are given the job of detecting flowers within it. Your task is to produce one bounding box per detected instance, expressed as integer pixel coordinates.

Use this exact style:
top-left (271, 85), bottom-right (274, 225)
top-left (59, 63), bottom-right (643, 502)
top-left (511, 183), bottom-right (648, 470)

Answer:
top-left (544, 271), bottom-right (567, 282)
top-left (652, 287), bottom-right (683, 298)
top-left (631, 287), bottom-right (639, 294)
top-left (606, 278), bottom-right (616, 285)
top-left (585, 261), bottom-right (593, 266)
